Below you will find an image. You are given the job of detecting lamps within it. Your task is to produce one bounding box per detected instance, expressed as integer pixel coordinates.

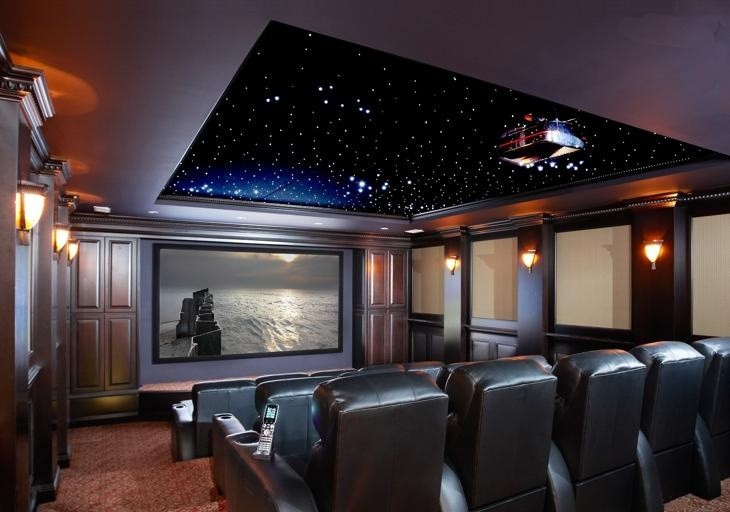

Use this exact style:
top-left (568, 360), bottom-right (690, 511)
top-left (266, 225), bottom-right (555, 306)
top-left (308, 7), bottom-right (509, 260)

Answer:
top-left (521, 249), bottom-right (537, 274)
top-left (67, 238), bottom-right (81, 261)
top-left (18, 179), bottom-right (50, 232)
top-left (445, 255), bottom-right (458, 275)
top-left (52, 222), bottom-right (71, 254)
top-left (642, 239), bottom-right (664, 270)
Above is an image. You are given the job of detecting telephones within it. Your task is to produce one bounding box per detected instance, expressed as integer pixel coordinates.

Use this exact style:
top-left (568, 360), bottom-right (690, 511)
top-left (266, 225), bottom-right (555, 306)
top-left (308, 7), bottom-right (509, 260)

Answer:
top-left (252, 403), bottom-right (280, 461)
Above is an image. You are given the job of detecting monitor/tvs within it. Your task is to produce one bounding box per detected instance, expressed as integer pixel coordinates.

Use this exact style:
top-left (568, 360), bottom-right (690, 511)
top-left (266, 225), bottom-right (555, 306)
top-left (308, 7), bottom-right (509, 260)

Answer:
top-left (151, 242), bottom-right (345, 364)
